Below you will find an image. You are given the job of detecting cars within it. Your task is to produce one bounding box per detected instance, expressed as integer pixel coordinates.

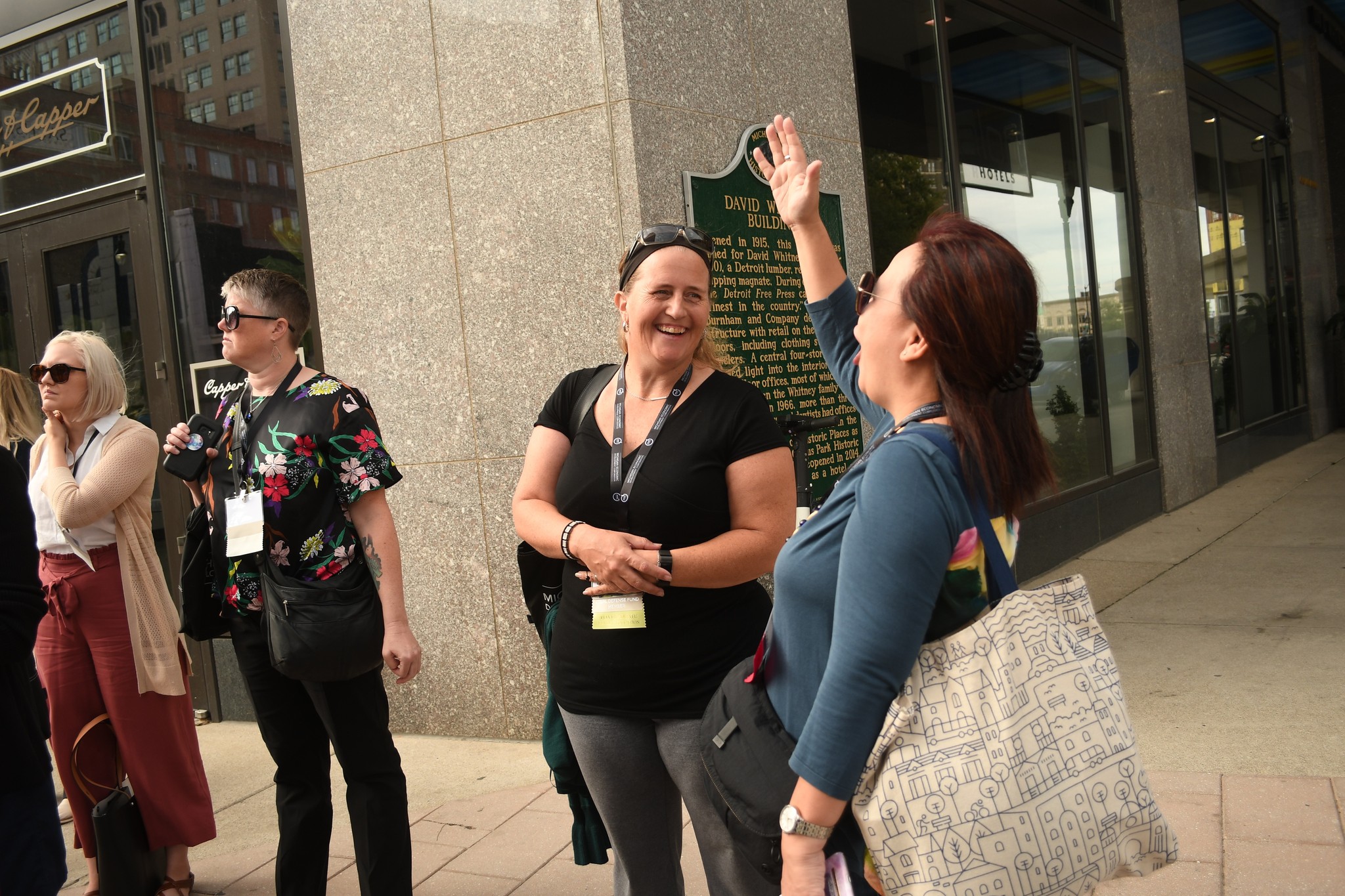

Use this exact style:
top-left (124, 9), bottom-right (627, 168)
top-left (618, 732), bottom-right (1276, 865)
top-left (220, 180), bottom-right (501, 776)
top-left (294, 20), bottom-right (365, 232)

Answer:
top-left (1028, 336), bottom-right (1129, 407)
top-left (1208, 333), bottom-right (1232, 355)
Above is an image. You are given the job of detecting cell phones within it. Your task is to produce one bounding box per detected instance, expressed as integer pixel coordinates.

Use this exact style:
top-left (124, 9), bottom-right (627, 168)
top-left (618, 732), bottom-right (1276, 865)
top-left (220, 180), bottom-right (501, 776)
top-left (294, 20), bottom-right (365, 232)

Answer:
top-left (164, 413), bottom-right (225, 482)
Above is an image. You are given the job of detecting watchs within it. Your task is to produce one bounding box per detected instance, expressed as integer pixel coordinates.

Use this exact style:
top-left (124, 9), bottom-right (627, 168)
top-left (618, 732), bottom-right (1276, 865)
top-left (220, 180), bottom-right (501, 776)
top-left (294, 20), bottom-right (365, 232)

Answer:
top-left (779, 803), bottom-right (834, 840)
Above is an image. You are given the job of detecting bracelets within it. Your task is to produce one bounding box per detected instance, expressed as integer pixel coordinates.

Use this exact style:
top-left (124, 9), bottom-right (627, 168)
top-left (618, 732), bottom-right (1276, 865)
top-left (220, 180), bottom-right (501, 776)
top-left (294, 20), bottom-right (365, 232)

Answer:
top-left (561, 520), bottom-right (585, 560)
top-left (656, 548), bottom-right (672, 591)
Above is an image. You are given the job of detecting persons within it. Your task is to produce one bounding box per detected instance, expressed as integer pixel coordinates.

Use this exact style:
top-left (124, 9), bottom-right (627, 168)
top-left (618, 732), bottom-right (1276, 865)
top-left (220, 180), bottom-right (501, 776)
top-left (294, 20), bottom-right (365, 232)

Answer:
top-left (164, 269), bottom-right (421, 896)
top-left (0, 367), bottom-right (68, 896)
top-left (511, 224), bottom-right (797, 896)
top-left (754, 114), bottom-right (1058, 896)
top-left (28, 330), bottom-right (216, 896)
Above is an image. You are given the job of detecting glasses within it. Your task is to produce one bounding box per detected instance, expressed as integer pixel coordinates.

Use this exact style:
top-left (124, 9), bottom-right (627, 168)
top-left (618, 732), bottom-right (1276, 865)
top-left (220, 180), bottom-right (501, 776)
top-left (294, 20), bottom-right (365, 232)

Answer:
top-left (855, 271), bottom-right (903, 316)
top-left (29, 363), bottom-right (86, 384)
top-left (220, 304), bottom-right (295, 335)
top-left (623, 223), bottom-right (715, 273)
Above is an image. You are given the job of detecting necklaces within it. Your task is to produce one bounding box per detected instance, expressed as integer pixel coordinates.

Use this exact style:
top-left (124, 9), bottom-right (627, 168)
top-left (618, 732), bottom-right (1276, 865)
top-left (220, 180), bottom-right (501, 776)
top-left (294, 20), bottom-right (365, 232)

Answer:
top-left (624, 384), bottom-right (669, 401)
top-left (246, 374), bottom-right (288, 419)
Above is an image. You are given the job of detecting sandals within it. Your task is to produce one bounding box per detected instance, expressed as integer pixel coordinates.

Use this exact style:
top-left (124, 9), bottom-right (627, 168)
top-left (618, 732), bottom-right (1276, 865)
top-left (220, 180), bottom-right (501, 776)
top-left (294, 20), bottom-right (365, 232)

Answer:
top-left (156, 870), bottom-right (194, 896)
top-left (82, 888), bottom-right (100, 896)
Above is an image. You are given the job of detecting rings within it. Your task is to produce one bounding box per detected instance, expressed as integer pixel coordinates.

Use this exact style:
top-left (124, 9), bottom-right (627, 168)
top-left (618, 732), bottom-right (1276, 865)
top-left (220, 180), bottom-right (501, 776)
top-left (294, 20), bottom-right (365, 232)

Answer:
top-left (784, 155), bottom-right (791, 161)
top-left (594, 574), bottom-right (602, 585)
top-left (166, 438), bottom-right (174, 445)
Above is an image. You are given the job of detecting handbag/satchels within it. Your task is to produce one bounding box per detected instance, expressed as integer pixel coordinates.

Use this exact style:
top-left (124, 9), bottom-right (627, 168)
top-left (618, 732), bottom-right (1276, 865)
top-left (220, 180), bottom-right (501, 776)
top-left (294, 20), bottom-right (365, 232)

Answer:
top-left (70, 712), bottom-right (157, 896)
top-left (172, 383), bottom-right (243, 642)
top-left (830, 435), bottom-right (1179, 896)
